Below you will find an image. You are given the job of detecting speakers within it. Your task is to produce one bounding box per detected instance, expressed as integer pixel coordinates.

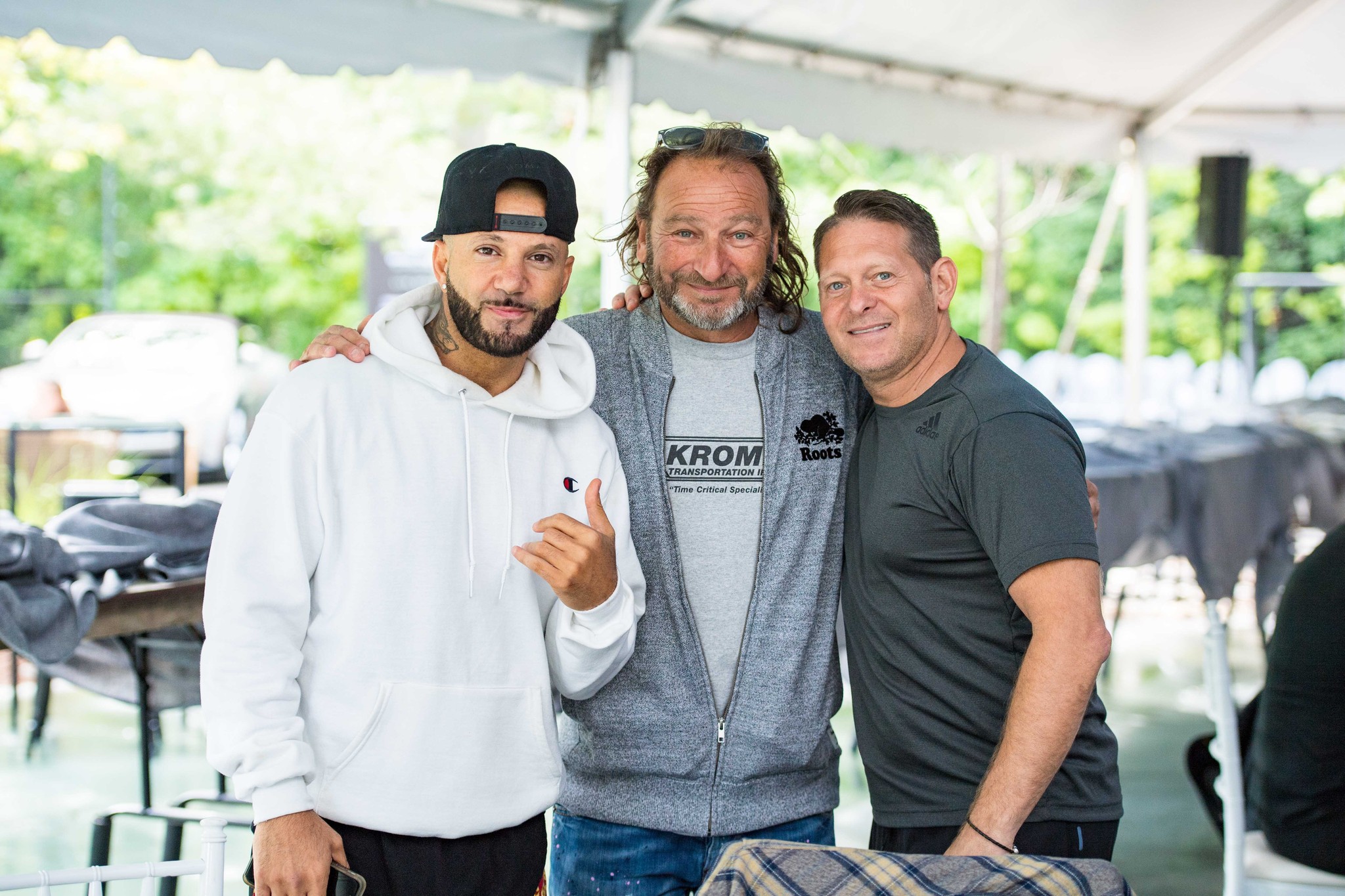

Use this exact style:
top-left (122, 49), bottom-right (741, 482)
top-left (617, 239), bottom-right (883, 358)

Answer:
top-left (1196, 156), bottom-right (1249, 257)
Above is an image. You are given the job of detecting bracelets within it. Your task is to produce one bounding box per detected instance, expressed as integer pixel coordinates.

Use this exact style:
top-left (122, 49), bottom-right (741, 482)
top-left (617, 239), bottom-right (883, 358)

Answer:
top-left (966, 816), bottom-right (1019, 855)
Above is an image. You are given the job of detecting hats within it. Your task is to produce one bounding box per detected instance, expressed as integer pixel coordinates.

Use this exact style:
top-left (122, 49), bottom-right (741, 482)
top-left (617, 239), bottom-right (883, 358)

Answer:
top-left (420, 143), bottom-right (579, 244)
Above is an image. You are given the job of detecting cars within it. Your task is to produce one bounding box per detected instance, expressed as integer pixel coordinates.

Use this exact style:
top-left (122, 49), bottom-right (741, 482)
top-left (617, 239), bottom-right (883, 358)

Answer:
top-left (0, 309), bottom-right (285, 478)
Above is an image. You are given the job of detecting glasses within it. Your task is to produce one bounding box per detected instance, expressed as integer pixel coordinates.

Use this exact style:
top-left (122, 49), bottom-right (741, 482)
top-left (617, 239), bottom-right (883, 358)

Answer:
top-left (655, 125), bottom-right (768, 154)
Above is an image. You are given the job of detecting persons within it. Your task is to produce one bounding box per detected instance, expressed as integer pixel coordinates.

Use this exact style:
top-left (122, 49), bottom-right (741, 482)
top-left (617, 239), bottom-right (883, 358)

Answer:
top-left (1241, 525), bottom-right (1345, 876)
top-left (290, 128), bottom-right (1101, 896)
top-left (200, 142), bottom-right (644, 896)
top-left (815, 189), bottom-right (1125, 863)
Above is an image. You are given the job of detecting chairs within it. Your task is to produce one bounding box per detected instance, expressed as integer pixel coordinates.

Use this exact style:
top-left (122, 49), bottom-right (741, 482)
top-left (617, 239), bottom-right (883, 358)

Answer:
top-left (1206, 595), bottom-right (1345, 893)
top-left (8, 423), bottom-right (199, 753)
top-left (695, 838), bottom-right (1127, 896)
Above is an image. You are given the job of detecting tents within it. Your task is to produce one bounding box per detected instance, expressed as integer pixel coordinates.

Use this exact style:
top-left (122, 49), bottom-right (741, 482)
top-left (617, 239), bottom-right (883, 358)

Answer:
top-left (0, 0), bottom-right (1345, 399)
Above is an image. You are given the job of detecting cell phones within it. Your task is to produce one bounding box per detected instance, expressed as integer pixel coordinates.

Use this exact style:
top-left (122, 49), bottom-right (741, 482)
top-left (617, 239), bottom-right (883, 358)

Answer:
top-left (243, 852), bottom-right (366, 896)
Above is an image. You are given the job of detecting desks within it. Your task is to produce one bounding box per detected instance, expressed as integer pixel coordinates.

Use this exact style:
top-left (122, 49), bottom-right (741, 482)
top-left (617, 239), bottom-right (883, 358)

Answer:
top-left (16, 551), bottom-right (213, 810)
top-left (1074, 424), bottom-right (1345, 582)
